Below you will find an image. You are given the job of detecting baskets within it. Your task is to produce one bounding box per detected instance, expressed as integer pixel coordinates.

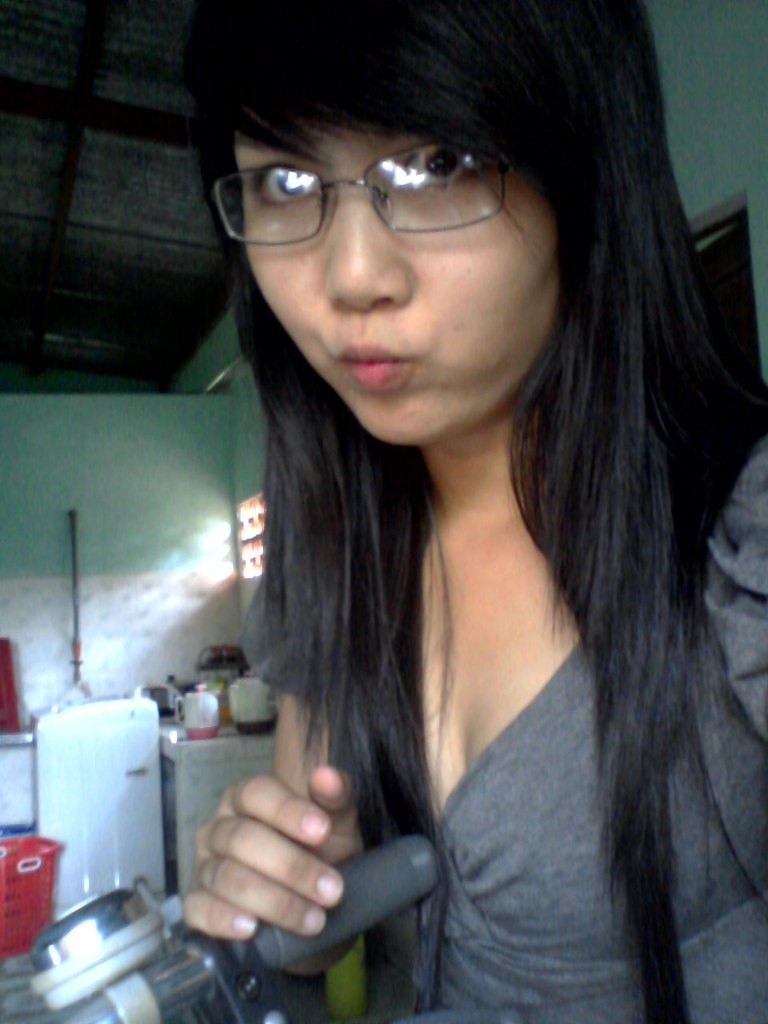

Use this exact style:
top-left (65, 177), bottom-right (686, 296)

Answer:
top-left (0, 837), bottom-right (58, 956)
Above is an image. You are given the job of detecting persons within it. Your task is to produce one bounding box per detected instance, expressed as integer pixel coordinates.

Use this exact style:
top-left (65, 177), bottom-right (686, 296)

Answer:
top-left (176, 0), bottom-right (768, 1024)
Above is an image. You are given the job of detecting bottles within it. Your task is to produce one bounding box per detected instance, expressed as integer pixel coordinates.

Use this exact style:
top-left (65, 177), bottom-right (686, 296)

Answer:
top-left (165, 675), bottom-right (179, 689)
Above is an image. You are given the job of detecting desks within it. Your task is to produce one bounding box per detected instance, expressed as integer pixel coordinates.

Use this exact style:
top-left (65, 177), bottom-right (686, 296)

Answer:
top-left (157, 717), bottom-right (276, 897)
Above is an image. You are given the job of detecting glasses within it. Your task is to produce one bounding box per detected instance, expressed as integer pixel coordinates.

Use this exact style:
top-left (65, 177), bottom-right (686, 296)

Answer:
top-left (211, 141), bottom-right (508, 246)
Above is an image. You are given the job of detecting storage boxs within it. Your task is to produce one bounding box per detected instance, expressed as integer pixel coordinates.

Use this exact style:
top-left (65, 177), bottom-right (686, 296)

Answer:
top-left (0, 835), bottom-right (63, 960)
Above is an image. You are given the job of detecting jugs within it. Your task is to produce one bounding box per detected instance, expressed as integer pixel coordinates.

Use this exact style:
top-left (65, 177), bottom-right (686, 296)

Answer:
top-left (229, 670), bottom-right (274, 734)
top-left (174, 685), bottom-right (220, 740)
